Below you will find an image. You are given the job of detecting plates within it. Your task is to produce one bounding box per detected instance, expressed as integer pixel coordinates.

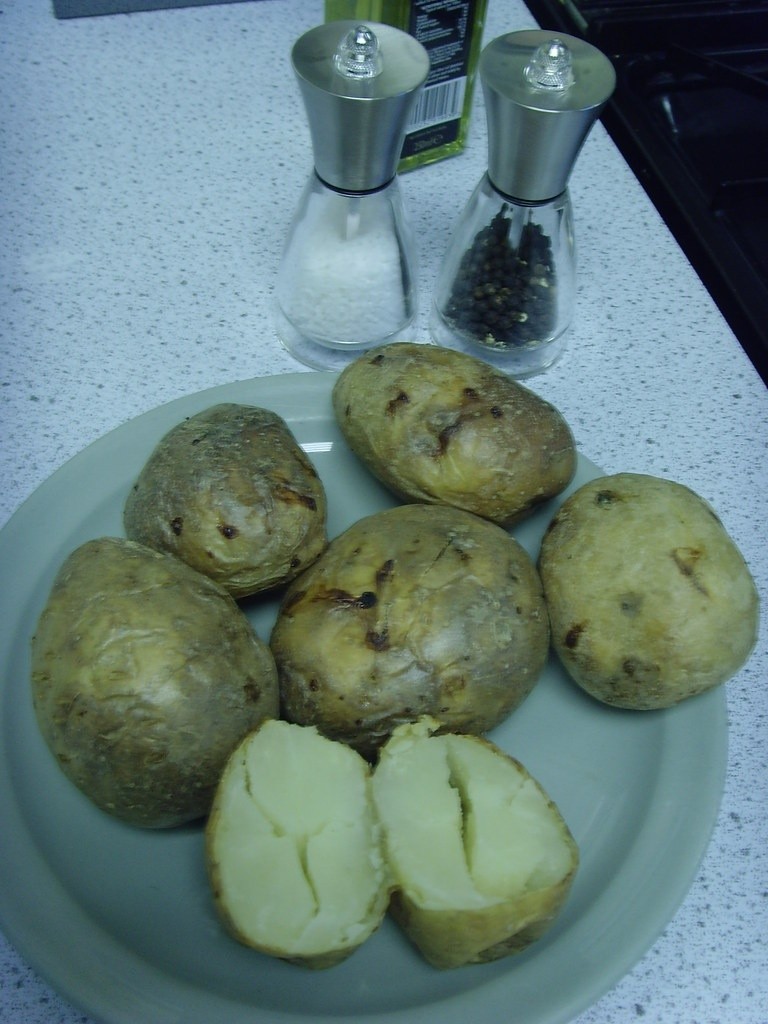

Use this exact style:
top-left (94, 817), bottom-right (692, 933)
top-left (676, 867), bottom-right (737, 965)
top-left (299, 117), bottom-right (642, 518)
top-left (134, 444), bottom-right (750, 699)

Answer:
top-left (1, 370), bottom-right (729, 1024)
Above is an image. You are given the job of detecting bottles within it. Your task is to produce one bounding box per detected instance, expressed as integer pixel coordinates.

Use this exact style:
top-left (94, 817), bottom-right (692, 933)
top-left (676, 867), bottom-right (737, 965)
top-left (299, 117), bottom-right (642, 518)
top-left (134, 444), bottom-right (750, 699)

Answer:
top-left (274, 23), bottom-right (430, 370)
top-left (424, 29), bottom-right (617, 375)
top-left (322, 0), bottom-right (489, 172)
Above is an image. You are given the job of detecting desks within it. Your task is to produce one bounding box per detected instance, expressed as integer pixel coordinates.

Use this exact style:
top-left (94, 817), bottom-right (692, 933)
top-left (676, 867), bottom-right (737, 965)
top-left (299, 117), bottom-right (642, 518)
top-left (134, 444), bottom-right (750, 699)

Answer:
top-left (1, 1), bottom-right (766, 1024)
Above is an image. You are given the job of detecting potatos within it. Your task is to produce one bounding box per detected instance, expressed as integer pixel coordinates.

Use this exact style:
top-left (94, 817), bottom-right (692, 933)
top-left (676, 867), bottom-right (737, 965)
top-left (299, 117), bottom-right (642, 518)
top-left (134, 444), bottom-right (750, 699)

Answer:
top-left (29, 341), bottom-right (760, 972)
